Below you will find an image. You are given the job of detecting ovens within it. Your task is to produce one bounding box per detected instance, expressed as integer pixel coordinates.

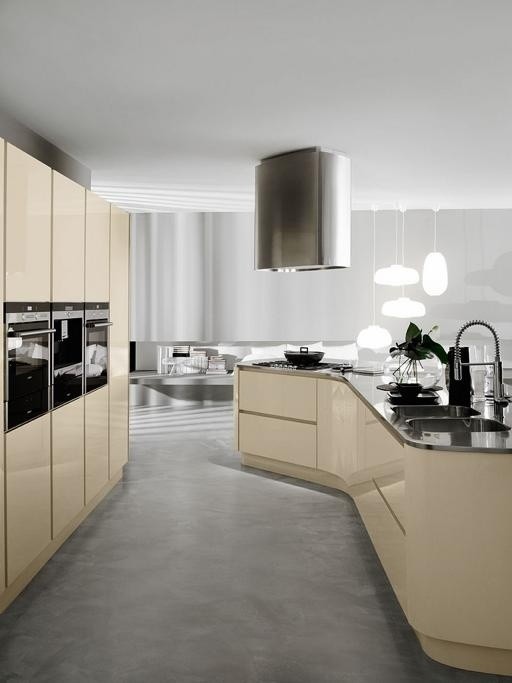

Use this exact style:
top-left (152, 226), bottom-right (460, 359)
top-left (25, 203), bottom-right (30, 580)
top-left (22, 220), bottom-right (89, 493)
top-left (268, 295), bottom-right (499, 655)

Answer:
top-left (3, 301), bottom-right (114, 435)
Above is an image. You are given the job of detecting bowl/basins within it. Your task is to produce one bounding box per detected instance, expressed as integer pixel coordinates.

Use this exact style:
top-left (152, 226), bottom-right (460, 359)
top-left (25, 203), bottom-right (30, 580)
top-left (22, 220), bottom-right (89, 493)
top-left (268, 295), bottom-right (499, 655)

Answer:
top-left (396, 383), bottom-right (423, 397)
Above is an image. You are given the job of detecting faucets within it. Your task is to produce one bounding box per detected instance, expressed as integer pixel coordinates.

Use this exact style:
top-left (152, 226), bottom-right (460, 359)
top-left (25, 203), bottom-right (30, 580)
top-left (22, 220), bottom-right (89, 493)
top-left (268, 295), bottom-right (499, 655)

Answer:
top-left (453, 320), bottom-right (509, 423)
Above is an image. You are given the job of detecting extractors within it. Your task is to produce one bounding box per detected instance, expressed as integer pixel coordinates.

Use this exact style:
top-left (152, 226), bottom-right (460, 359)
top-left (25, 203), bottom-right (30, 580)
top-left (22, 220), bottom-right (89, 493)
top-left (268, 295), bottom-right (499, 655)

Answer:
top-left (252, 144), bottom-right (354, 273)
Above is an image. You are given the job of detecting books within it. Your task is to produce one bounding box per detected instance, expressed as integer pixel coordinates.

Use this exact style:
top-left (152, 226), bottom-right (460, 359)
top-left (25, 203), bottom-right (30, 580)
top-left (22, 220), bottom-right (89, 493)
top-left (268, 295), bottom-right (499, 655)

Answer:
top-left (173, 346), bottom-right (228, 374)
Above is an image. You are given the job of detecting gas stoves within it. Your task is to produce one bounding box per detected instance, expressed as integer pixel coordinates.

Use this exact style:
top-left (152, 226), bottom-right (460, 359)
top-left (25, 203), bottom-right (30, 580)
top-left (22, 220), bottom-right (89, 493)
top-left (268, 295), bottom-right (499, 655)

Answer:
top-left (252, 360), bottom-right (353, 371)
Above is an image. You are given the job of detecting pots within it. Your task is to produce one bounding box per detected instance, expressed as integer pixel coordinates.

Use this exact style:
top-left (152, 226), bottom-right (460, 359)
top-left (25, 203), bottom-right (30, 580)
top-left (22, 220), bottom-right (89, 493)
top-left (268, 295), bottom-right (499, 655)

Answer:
top-left (284, 346), bottom-right (326, 366)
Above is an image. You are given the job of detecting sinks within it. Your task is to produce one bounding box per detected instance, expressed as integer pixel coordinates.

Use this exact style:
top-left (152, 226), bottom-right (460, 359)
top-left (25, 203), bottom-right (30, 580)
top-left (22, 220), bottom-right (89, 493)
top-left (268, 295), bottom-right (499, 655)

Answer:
top-left (391, 404), bottom-right (483, 418)
top-left (400, 418), bottom-right (512, 434)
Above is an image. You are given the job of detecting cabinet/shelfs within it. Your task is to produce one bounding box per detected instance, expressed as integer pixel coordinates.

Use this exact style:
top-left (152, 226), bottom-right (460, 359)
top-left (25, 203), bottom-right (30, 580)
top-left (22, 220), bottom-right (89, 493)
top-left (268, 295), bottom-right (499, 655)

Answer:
top-left (235, 368), bottom-right (317, 490)
top-left (111, 204), bottom-right (131, 486)
top-left (0, 136), bottom-right (110, 301)
top-left (3, 383), bottom-right (109, 610)
top-left (371, 411), bottom-right (407, 627)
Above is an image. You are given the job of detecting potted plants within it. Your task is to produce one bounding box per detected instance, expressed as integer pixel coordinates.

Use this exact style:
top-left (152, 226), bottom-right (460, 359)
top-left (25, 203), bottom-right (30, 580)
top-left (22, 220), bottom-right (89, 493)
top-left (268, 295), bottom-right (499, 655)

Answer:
top-left (389, 321), bottom-right (449, 390)
